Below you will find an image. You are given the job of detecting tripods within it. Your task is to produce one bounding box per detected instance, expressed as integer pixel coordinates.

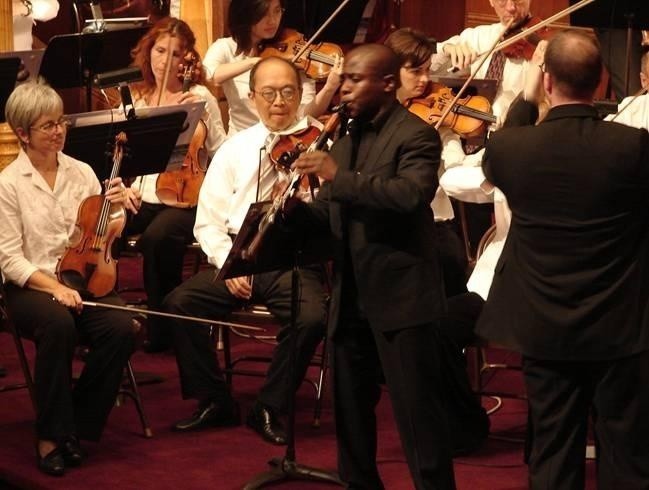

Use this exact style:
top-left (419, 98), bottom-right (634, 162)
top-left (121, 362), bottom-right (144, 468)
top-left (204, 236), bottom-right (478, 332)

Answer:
top-left (239, 265), bottom-right (350, 490)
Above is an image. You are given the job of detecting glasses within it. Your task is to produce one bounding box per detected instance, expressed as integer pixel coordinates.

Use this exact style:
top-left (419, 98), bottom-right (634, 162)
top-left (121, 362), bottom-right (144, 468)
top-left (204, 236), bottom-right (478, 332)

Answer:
top-left (255, 86), bottom-right (295, 101)
top-left (31, 116), bottom-right (74, 133)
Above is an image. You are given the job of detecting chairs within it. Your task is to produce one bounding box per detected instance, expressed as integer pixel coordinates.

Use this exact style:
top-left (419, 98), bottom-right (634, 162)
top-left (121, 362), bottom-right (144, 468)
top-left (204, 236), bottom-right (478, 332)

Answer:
top-left (212, 308), bottom-right (332, 432)
top-left (470, 338), bottom-right (529, 426)
top-left (2, 304), bottom-right (152, 444)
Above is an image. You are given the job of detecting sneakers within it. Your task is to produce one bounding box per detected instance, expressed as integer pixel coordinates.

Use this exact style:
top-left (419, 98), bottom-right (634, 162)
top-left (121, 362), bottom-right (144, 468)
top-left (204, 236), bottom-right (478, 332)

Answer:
top-left (453, 408), bottom-right (491, 456)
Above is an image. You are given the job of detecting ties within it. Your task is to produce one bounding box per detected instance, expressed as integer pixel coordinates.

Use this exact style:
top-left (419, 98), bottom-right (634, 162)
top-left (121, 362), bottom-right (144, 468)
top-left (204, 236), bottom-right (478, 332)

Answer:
top-left (460, 35), bottom-right (506, 154)
top-left (258, 134), bottom-right (280, 205)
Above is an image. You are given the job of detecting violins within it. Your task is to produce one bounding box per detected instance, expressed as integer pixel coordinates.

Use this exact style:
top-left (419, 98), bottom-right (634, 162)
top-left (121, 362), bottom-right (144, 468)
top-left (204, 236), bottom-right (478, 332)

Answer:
top-left (154, 48), bottom-right (209, 209)
top-left (500, 16), bottom-right (561, 62)
top-left (257, 28), bottom-right (349, 81)
top-left (400, 83), bottom-right (498, 141)
top-left (57, 133), bottom-right (137, 300)
top-left (269, 127), bottom-right (330, 198)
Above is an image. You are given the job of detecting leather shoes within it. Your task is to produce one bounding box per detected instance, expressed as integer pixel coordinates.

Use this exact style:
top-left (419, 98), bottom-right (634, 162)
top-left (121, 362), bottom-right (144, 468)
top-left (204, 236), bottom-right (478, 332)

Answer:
top-left (36, 436), bottom-right (83, 475)
top-left (142, 335), bottom-right (168, 353)
top-left (247, 403), bottom-right (287, 446)
top-left (174, 401), bottom-right (226, 431)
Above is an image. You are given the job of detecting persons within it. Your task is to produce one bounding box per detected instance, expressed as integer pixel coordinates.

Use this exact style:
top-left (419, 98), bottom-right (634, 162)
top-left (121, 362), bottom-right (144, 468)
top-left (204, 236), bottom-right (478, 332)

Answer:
top-left (601, 50), bottom-right (648, 130)
top-left (123, 2), bottom-right (550, 457)
top-left (1, 82), bottom-right (136, 479)
top-left (271, 44), bottom-right (465, 489)
top-left (481, 31), bottom-right (648, 489)
